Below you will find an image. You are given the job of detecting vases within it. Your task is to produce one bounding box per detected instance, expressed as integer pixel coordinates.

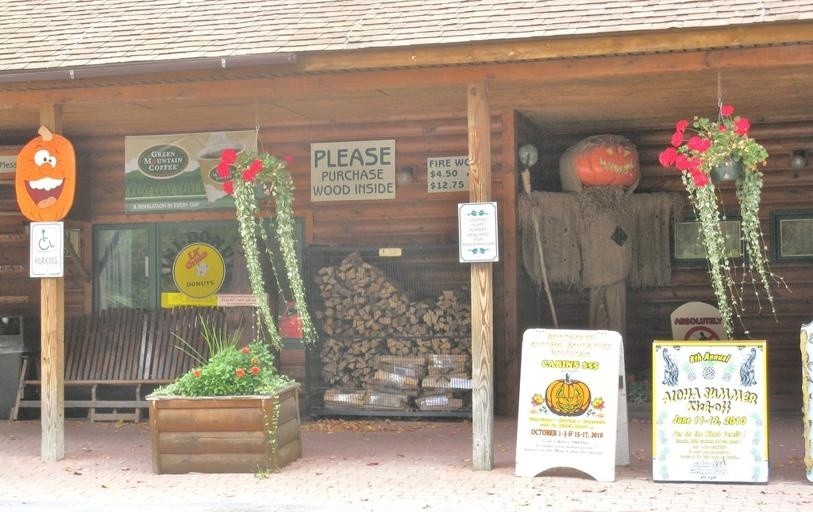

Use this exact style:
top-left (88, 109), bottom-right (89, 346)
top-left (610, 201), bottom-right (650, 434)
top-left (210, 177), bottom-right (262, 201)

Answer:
top-left (252, 177), bottom-right (268, 198)
top-left (711, 154), bottom-right (741, 182)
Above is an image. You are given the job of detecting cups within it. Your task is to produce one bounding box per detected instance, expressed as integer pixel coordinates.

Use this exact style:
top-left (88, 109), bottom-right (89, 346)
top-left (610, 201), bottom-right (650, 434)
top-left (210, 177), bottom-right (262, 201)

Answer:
top-left (197, 141), bottom-right (248, 202)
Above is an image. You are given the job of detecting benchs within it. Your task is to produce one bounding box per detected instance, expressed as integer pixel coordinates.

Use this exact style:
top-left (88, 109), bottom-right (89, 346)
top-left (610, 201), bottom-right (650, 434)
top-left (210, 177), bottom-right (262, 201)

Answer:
top-left (8, 303), bottom-right (258, 425)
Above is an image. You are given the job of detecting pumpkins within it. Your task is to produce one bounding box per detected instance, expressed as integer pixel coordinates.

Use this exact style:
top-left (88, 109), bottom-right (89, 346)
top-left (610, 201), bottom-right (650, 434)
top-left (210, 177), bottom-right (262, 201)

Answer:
top-left (545, 374), bottom-right (592, 416)
top-left (15, 126), bottom-right (77, 221)
top-left (574, 139), bottom-right (639, 186)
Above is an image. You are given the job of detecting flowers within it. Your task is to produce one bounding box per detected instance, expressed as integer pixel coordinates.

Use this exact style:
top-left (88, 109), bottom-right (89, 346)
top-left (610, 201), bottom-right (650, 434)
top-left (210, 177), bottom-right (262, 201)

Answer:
top-left (215, 147), bottom-right (320, 353)
top-left (655, 105), bottom-right (790, 341)
top-left (151, 344), bottom-right (293, 397)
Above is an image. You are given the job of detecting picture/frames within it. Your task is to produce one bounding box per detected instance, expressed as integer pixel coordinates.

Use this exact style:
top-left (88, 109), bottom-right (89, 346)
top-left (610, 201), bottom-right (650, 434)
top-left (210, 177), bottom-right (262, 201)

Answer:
top-left (667, 215), bottom-right (748, 274)
top-left (771, 208), bottom-right (812, 267)
